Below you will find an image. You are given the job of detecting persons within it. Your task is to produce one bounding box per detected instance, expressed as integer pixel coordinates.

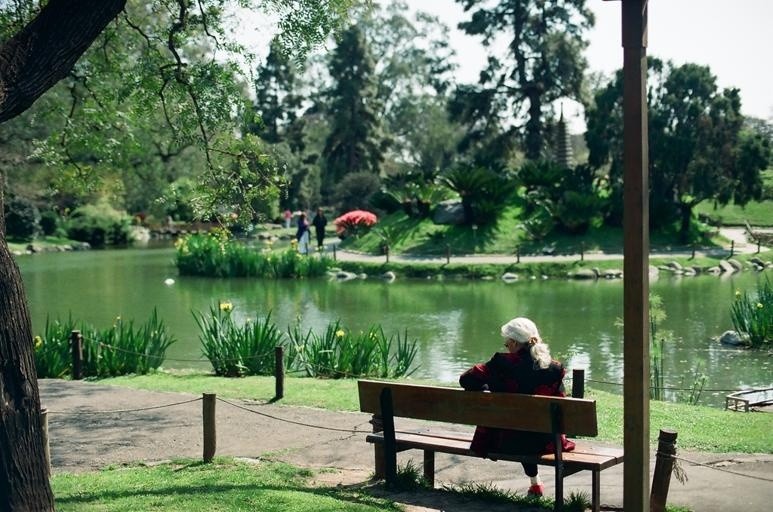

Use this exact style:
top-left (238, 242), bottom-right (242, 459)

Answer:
top-left (454, 316), bottom-right (577, 496)
top-left (311, 208), bottom-right (327, 249)
top-left (283, 206), bottom-right (290, 228)
top-left (295, 212), bottom-right (310, 255)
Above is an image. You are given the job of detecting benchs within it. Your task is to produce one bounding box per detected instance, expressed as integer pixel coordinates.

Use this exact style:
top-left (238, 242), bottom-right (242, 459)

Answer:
top-left (356, 379), bottom-right (625, 512)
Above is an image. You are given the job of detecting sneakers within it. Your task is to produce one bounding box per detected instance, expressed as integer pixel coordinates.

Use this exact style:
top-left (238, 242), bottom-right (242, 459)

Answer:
top-left (528, 484), bottom-right (544, 496)
